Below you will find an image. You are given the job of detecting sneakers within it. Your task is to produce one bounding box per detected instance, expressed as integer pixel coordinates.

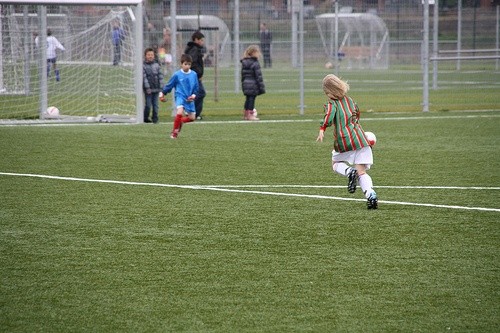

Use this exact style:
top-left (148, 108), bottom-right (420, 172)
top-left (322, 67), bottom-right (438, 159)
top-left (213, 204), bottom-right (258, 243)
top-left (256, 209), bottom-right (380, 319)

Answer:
top-left (171, 130), bottom-right (178, 139)
top-left (348, 168), bottom-right (359, 193)
top-left (367, 193), bottom-right (378, 210)
top-left (144, 116), bottom-right (158, 123)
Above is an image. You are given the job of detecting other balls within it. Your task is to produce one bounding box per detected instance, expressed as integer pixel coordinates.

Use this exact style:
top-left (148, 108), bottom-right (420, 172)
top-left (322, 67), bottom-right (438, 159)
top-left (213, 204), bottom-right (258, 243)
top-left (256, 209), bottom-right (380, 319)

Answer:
top-left (325, 62), bottom-right (333, 68)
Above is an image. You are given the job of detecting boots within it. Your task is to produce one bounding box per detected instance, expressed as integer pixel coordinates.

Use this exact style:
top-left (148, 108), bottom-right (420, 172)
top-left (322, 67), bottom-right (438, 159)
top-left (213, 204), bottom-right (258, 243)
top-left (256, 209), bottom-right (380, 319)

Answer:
top-left (244, 108), bottom-right (259, 121)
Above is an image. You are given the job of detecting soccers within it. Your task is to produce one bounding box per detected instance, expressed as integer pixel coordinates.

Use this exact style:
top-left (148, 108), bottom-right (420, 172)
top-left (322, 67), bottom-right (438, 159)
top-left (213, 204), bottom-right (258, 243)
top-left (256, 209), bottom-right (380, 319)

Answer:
top-left (364, 132), bottom-right (376, 145)
top-left (47, 106), bottom-right (59, 115)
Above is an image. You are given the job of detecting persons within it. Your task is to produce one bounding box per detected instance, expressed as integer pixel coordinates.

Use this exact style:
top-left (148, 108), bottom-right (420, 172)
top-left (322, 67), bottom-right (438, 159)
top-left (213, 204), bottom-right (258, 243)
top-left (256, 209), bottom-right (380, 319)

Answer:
top-left (33, 31), bottom-right (39, 48)
top-left (316, 74), bottom-right (378, 209)
top-left (161, 28), bottom-right (172, 64)
top-left (184, 32), bottom-right (205, 120)
top-left (113, 23), bottom-right (124, 66)
top-left (159, 55), bottom-right (199, 139)
top-left (240, 46), bottom-right (266, 121)
top-left (46, 28), bottom-right (66, 82)
top-left (259, 22), bottom-right (272, 68)
top-left (143, 48), bottom-right (163, 123)
top-left (148, 20), bottom-right (159, 60)
